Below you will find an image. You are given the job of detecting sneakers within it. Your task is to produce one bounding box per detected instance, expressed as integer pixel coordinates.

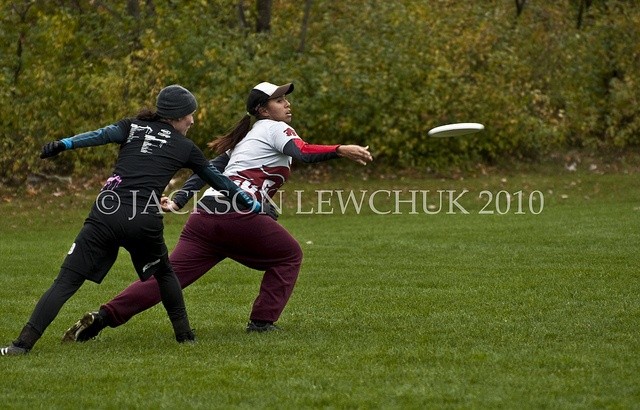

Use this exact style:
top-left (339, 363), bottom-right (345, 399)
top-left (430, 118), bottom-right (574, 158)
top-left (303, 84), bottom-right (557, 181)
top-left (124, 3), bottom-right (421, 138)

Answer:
top-left (176, 331), bottom-right (196, 343)
top-left (1, 344), bottom-right (28, 356)
top-left (245, 319), bottom-right (282, 334)
top-left (60, 310), bottom-right (103, 343)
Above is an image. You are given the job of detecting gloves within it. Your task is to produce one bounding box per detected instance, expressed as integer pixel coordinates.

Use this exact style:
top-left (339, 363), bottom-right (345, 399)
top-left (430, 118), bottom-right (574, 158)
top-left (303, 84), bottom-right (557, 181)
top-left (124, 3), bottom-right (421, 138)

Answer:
top-left (258, 201), bottom-right (278, 222)
top-left (38, 139), bottom-right (65, 159)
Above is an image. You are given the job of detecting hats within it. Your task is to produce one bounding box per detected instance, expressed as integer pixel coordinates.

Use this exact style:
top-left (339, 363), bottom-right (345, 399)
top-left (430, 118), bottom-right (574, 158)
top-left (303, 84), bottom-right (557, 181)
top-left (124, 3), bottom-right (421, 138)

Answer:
top-left (246, 82), bottom-right (294, 113)
top-left (156, 83), bottom-right (198, 121)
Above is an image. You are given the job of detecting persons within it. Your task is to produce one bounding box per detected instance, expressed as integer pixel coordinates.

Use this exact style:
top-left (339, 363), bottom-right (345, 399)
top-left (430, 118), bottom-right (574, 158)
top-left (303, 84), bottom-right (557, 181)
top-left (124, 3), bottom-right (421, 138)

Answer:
top-left (0, 85), bottom-right (279, 358)
top-left (60, 81), bottom-right (374, 345)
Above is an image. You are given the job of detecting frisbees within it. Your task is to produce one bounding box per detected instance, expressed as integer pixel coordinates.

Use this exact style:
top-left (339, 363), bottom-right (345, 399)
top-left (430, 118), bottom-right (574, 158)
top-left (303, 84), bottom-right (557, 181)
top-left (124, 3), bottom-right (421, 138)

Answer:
top-left (427, 121), bottom-right (485, 138)
